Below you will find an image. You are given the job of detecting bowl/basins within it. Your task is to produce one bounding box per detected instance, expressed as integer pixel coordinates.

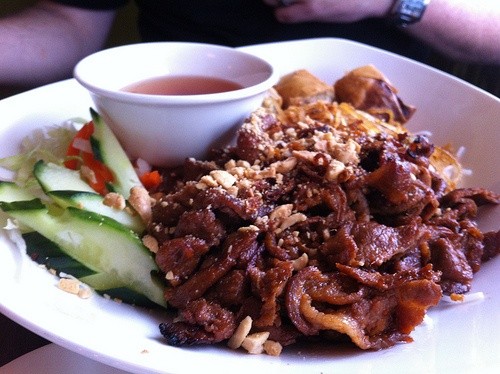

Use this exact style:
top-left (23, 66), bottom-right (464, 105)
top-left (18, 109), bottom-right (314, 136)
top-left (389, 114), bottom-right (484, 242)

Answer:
top-left (0, 38), bottom-right (500, 374)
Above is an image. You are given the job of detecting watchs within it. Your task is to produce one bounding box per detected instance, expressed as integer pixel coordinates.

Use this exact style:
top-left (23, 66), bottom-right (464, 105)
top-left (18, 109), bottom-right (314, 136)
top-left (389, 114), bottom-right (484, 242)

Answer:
top-left (385, 0), bottom-right (431, 32)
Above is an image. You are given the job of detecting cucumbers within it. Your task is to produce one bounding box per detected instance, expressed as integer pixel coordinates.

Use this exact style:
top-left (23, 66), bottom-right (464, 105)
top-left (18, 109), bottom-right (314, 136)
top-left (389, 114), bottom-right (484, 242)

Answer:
top-left (0, 107), bottom-right (169, 309)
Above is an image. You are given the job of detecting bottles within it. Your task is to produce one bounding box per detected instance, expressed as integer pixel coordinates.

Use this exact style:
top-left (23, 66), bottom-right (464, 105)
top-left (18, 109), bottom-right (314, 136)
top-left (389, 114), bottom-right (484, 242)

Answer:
top-left (392, 0), bottom-right (428, 30)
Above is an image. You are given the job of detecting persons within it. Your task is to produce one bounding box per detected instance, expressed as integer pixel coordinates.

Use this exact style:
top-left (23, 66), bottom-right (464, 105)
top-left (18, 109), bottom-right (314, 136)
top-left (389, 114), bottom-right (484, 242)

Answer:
top-left (0, 0), bottom-right (500, 96)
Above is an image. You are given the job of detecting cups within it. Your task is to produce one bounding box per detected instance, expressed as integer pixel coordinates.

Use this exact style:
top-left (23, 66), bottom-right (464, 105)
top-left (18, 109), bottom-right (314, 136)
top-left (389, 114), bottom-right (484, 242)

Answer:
top-left (74, 42), bottom-right (279, 167)
